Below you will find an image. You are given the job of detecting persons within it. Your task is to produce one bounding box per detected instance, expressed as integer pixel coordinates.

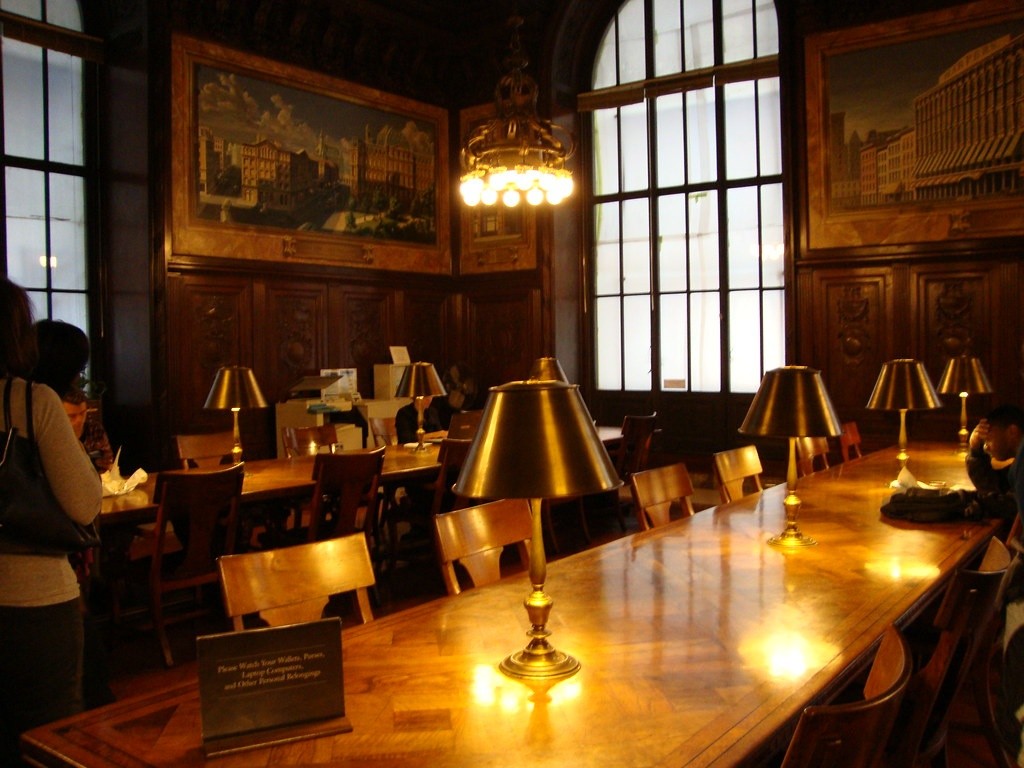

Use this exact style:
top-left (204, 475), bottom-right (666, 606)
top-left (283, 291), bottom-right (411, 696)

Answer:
top-left (0, 278), bottom-right (105, 768)
top-left (965, 404), bottom-right (1024, 527)
top-left (18, 320), bottom-right (114, 712)
top-left (395, 393), bottom-right (443, 445)
top-left (59, 388), bottom-right (113, 474)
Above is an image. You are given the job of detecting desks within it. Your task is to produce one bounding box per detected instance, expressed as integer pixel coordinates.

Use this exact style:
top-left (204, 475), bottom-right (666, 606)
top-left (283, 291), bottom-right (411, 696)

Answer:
top-left (20, 442), bottom-right (1012, 767)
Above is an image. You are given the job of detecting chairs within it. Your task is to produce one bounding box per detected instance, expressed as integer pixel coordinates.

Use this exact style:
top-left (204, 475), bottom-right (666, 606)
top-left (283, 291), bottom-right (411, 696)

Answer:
top-left (125, 428), bottom-right (1024, 768)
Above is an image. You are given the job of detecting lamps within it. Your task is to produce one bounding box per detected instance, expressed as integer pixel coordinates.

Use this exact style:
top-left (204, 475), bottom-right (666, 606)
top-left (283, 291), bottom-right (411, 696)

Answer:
top-left (396, 361), bottom-right (447, 450)
top-left (528, 356), bottom-right (571, 381)
top-left (101, 428), bottom-right (623, 615)
top-left (459, 0), bottom-right (575, 209)
top-left (203, 367), bottom-right (268, 466)
top-left (736, 366), bottom-right (843, 547)
top-left (450, 378), bottom-right (623, 677)
top-left (935, 357), bottom-right (993, 447)
top-left (866, 357), bottom-right (941, 490)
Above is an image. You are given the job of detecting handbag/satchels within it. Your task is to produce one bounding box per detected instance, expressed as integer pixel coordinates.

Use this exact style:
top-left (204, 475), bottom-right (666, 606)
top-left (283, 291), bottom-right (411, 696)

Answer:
top-left (880, 487), bottom-right (981, 522)
top-left (0, 375), bottom-right (103, 555)
